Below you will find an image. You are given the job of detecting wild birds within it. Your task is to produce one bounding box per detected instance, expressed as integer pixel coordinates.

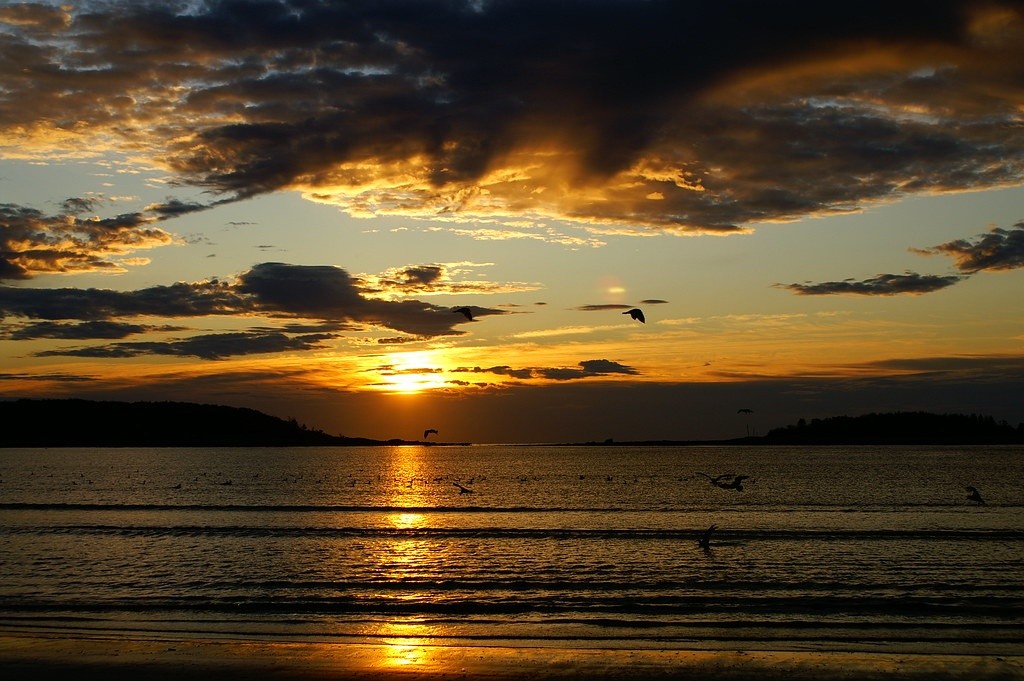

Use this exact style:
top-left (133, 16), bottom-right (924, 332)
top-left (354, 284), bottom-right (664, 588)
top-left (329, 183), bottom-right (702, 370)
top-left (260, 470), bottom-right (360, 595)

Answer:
top-left (424, 429), bottom-right (438, 438)
top-left (696, 525), bottom-right (717, 544)
top-left (718, 475), bottom-right (750, 491)
top-left (454, 483), bottom-right (474, 496)
top-left (622, 308), bottom-right (647, 326)
top-left (694, 470), bottom-right (736, 486)
top-left (736, 408), bottom-right (753, 414)
top-left (965, 485), bottom-right (986, 504)
top-left (453, 307), bottom-right (474, 321)
